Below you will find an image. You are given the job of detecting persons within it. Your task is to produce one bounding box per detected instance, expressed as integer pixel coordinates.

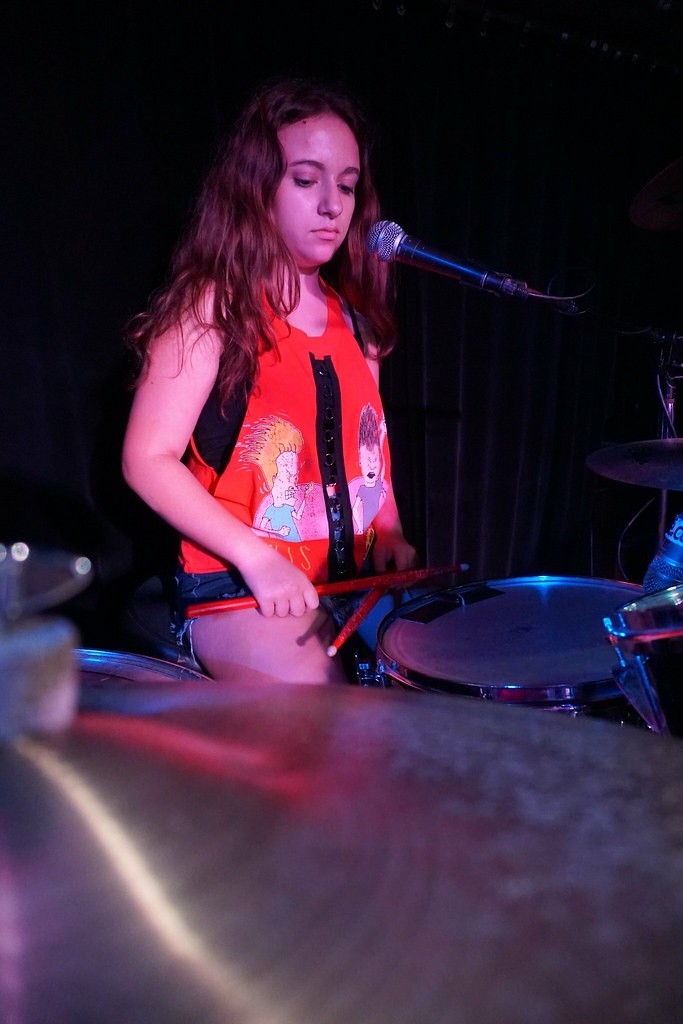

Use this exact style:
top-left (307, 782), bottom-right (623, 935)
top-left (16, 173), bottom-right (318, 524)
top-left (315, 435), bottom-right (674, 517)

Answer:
top-left (123, 85), bottom-right (439, 684)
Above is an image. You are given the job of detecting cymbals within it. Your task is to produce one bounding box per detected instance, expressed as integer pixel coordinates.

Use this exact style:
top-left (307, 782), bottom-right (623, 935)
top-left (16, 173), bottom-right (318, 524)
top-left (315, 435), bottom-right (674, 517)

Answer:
top-left (0, 679), bottom-right (683, 1024)
top-left (583, 437), bottom-right (683, 492)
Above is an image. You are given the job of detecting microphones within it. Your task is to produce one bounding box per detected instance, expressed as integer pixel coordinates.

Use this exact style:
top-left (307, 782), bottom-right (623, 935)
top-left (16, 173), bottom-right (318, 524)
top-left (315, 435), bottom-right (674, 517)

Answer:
top-left (365, 220), bottom-right (544, 301)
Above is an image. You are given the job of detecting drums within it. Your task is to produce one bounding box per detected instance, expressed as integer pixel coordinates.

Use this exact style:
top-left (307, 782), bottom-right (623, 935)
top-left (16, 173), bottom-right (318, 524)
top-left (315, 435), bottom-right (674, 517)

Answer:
top-left (70, 647), bottom-right (216, 683)
top-left (372, 573), bottom-right (651, 733)
top-left (601, 583), bottom-right (683, 740)
